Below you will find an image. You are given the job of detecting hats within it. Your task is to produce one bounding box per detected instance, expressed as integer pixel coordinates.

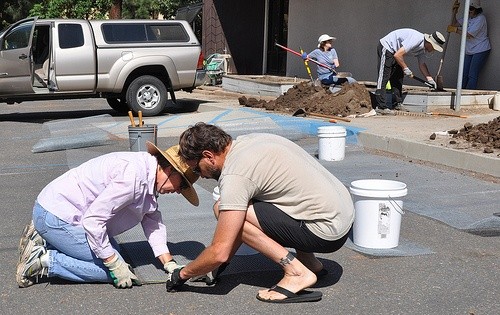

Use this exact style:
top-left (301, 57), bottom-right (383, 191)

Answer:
top-left (469, 0), bottom-right (481, 8)
top-left (317, 34), bottom-right (336, 48)
top-left (145, 139), bottom-right (202, 207)
top-left (425, 31), bottom-right (445, 52)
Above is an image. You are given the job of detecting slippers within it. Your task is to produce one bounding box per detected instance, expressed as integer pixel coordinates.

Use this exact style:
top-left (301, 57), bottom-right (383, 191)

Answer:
top-left (314, 269), bottom-right (328, 278)
top-left (256, 283), bottom-right (322, 303)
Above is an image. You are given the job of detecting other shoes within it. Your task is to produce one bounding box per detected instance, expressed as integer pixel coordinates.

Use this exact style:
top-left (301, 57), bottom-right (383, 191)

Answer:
top-left (395, 103), bottom-right (409, 112)
top-left (376, 109), bottom-right (390, 114)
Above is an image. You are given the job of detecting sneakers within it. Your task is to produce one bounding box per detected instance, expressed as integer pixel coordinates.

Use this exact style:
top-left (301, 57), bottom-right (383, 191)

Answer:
top-left (16, 220), bottom-right (47, 288)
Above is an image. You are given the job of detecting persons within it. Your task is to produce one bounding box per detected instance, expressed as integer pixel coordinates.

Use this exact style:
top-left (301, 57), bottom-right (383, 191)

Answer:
top-left (447, 0), bottom-right (492, 90)
top-left (375, 28), bottom-right (446, 113)
top-left (16, 139), bottom-right (201, 289)
top-left (302, 34), bottom-right (357, 87)
top-left (166, 121), bottom-right (355, 304)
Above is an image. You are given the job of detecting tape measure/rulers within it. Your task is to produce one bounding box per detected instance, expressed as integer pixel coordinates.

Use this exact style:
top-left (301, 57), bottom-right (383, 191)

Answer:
top-left (141, 269), bottom-right (281, 284)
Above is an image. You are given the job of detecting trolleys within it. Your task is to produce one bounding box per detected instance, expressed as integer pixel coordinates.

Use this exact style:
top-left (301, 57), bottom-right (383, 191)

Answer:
top-left (206, 53), bottom-right (226, 85)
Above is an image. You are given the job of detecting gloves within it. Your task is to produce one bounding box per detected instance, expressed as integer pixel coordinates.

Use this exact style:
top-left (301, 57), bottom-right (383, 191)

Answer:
top-left (166, 265), bottom-right (190, 292)
top-left (103, 250), bottom-right (141, 288)
top-left (301, 52), bottom-right (307, 60)
top-left (403, 67), bottom-right (412, 76)
top-left (451, 0), bottom-right (460, 14)
top-left (164, 259), bottom-right (186, 273)
top-left (329, 66), bottom-right (336, 72)
top-left (427, 79), bottom-right (437, 90)
top-left (190, 262), bottom-right (227, 285)
top-left (447, 25), bottom-right (458, 33)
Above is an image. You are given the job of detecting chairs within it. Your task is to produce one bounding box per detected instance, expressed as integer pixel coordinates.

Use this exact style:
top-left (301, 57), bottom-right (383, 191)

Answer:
top-left (34, 35), bottom-right (48, 68)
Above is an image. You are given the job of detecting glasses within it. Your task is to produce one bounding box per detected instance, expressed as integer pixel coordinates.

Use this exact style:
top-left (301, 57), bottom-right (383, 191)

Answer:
top-left (180, 175), bottom-right (187, 189)
top-left (193, 157), bottom-right (201, 176)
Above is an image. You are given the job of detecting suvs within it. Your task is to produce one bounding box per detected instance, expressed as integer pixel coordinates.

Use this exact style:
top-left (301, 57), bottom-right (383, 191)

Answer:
top-left (0, 15), bottom-right (209, 116)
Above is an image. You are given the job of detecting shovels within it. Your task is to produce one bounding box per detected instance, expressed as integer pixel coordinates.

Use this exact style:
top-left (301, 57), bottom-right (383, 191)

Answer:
top-left (429, 0), bottom-right (458, 90)
top-left (275, 43), bottom-right (353, 77)
top-left (293, 108), bottom-right (351, 122)
top-left (299, 46), bottom-right (321, 86)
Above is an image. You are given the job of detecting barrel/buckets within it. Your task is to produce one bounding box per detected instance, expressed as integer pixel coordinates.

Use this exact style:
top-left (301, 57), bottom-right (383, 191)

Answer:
top-left (348, 178), bottom-right (408, 250)
top-left (129, 124), bottom-right (159, 152)
top-left (318, 125), bottom-right (347, 162)
top-left (493, 92), bottom-right (500, 110)
